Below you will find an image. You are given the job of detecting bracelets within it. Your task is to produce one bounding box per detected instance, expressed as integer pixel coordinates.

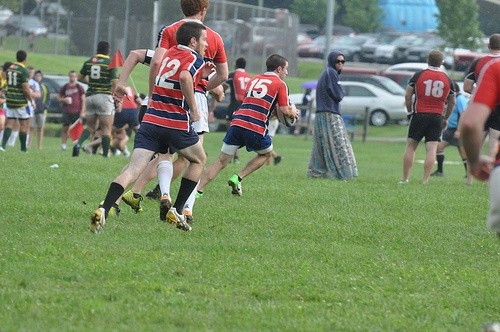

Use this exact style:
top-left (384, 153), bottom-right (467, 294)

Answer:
top-left (32, 100), bottom-right (34, 104)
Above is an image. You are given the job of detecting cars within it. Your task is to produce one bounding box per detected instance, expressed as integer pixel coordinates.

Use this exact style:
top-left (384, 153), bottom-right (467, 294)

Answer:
top-left (4, 14), bottom-right (48, 37)
top-left (338, 75), bottom-right (416, 95)
top-left (0, 5), bottom-right (14, 27)
top-left (41, 75), bottom-right (87, 113)
top-left (288, 82), bottom-right (408, 127)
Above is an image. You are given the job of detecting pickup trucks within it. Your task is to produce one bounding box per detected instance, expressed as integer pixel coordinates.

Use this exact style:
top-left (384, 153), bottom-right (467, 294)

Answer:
top-left (381, 63), bottom-right (477, 92)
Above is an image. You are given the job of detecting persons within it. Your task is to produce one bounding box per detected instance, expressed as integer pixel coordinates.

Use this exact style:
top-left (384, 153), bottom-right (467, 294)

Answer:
top-left (98, 1), bottom-right (229, 224)
top-left (400, 49), bottom-right (456, 185)
top-left (58, 70), bottom-right (86, 151)
top-left (0, 50), bottom-right (37, 153)
top-left (307, 50), bottom-right (358, 181)
top-left (89, 22), bottom-right (207, 233)
top-left (463, 34), bottom-right (500, 185)
top-left (459, 56), bottom-right (500, 237)
top-left (0, 62), bottom-right (313, 168)
top-left (197, 54), bottom-right (298, 200)
top-left (430, 82), bottom-right (468, 178)
top-left (72, 41), bottom-right (117, 155)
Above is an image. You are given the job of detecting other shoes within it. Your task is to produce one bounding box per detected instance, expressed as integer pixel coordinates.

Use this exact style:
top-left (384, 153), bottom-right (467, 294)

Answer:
top-left (431, 171), bottom-right (443, 176)
top-left (73, 144), bottom-right (80, 156)
top-left (275, 156), bottom-right (281, 163)
top-left (0, 147), bottom-right (7, 152)
top-left (99, 146), bottom-right (130, 157)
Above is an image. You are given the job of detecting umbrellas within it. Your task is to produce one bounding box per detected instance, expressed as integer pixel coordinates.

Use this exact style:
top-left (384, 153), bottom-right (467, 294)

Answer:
top-left (303, 80), bottom-right (317, 89)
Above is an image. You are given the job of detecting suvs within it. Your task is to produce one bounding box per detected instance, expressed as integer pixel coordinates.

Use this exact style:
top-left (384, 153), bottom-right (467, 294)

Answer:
top-left (340, 67), bottom-right (415, 90)
top-left (206, 18), bottom-right (499, 72)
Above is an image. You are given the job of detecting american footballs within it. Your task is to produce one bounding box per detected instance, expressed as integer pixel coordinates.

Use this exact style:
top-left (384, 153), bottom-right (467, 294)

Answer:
top-left (276, 96), bottom-right (297, 127)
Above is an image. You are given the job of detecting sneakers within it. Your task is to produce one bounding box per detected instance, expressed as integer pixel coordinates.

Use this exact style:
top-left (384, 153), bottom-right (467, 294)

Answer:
top-left (167, 206), bottom-right (192, 232)
top-left (228, 174), bottom-right (242, 197)
top-left (146, 186), bottom-right (161, 198)
top-left (98, 200), bottom-right (120, 219)
top-left (89, 207), bottom-right (105, 234)
top-left (160, 195), bottom-right (172, 220)
top-left (196, 192), bottom-right (203, 199)
top-left (182, 209), bottom-right (193, 221)
top-left (123, 190), bottom-right (144, 213)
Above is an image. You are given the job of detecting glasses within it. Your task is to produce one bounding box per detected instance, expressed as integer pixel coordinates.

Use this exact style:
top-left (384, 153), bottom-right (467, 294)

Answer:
top-left (336, 59), bottom-right (345, 64)
